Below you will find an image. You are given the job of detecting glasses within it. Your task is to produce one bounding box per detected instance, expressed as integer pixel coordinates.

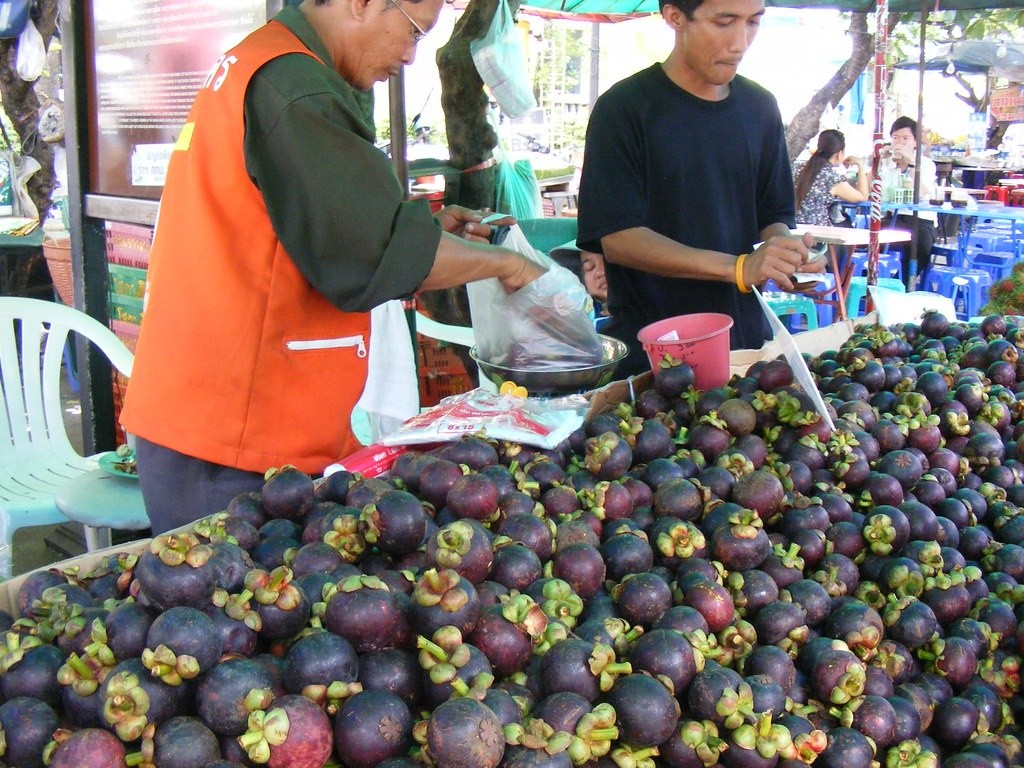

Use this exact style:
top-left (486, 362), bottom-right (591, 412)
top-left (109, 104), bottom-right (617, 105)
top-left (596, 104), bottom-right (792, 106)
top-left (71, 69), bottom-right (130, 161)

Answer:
top-left (890, 136), bottom-right (913, 144)
top-left (392, 0), bottom-right (427, 46)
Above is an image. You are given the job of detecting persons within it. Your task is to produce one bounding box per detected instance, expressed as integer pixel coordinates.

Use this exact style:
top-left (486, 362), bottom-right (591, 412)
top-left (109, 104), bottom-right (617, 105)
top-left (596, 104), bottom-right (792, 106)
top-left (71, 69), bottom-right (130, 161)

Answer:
top-left (866, 116), bottom-right (938, 293)
top-left (549, 238), bottom-right (615, 335)
top-left (573, 0), bottom-right (829, 384)
top-left (789, 129), bottom-right (870, 276)
top-left (118, 0), bottom-right (546, 540)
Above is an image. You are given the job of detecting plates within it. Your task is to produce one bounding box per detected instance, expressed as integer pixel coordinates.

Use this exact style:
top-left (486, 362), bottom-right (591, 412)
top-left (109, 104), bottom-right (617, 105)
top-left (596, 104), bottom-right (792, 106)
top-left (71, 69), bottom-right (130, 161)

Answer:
top-left (98, 448), bottom-right (138, 479)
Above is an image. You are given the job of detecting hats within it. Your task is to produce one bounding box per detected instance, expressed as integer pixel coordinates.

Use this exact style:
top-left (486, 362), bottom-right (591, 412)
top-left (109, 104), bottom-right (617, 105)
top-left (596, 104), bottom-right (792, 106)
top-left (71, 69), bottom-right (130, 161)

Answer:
top-left (549, 239), bottom-right (585, 275)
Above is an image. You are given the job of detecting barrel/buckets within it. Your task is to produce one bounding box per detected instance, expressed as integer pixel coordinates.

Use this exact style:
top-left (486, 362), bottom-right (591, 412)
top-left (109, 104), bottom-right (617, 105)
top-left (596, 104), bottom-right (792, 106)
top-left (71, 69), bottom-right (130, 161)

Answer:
top-left (637, 313), bottom-right (734, 391)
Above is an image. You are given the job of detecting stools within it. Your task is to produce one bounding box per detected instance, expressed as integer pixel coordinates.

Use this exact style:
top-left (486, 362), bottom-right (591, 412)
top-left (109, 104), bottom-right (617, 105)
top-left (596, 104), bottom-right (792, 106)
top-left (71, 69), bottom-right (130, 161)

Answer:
top-left (757, 183), bottom-right (1023, 336)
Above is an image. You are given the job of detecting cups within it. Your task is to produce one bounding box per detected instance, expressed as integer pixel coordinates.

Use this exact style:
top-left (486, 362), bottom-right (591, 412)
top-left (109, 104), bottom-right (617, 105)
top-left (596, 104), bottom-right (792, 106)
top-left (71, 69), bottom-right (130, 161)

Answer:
top-left (929, 189), bottom-right (945, 207)
top-left (892, 142), bottom-right (904, 162)
top-left (951, 190), bottom-right (968, 209)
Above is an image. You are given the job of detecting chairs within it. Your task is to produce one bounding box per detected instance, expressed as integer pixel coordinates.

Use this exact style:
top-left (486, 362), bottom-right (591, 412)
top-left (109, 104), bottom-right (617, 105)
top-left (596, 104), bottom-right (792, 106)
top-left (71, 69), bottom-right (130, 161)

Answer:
top-left (0, 295), bottom-right (146, 586)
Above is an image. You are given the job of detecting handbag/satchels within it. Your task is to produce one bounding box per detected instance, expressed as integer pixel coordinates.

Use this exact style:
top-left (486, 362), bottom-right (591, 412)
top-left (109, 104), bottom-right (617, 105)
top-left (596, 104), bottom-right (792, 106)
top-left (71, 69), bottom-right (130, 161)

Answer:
top-left (469, 0), bottom-right (538, 119)
top-left (828, 204), bottom-right (846, 224)
top-left (495, 159), bottom-right (543, 219)
top-left (467, 213), bottom-right (604, 371)
top-left (0, 0), bottom-right (46, 82)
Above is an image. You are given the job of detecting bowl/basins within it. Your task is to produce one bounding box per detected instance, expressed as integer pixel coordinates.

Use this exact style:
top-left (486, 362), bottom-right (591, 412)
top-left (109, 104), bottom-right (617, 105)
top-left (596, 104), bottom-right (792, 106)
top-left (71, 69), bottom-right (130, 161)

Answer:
top-left (469, 333), bottom-right (631, 398)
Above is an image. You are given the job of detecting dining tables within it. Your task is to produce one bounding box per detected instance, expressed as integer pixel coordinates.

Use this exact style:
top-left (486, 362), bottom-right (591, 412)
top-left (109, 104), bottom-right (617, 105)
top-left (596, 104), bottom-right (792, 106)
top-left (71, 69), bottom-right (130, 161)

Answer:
top-left (783, 222), bottom-right (911, 323)
top-left (829, 197), bottom-right (1024, 271)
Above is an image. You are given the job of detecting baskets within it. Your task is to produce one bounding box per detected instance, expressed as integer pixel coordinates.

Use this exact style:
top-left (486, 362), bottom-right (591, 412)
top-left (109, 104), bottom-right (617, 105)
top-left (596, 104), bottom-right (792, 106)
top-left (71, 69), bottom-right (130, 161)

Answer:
top-left (890, 187), bottom-right (915, 205)
top-left (41, 233), bottom-right (75, 307)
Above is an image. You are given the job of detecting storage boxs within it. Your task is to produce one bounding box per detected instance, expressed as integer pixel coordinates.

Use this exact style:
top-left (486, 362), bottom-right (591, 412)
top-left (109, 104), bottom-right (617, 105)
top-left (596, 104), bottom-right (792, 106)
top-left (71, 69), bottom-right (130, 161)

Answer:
top-left (96, 222), bottom-right (155, 449)
top-left (414, 331), bottom-right (475, 406)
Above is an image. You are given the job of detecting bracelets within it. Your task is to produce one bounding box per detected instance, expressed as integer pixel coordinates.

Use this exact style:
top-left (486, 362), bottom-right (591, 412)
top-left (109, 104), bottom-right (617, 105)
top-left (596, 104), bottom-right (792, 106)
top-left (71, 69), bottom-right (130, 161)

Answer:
top-left (735, 253), bottom-right (753, 295)
